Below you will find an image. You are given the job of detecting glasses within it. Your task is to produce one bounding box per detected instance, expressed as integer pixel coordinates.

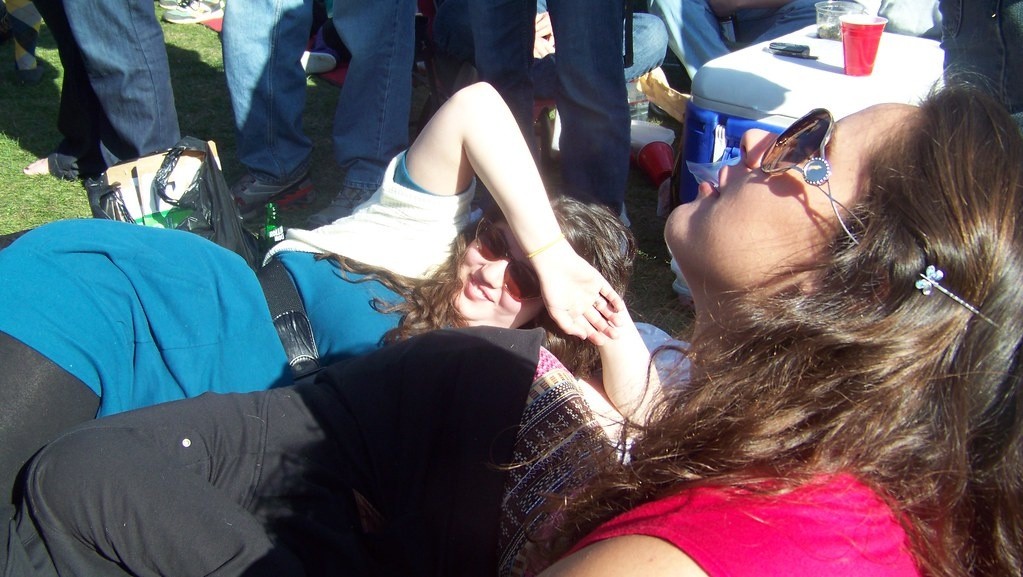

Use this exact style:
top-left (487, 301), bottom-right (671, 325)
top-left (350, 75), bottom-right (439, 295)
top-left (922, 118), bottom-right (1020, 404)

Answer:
top-left (475, 214), bottom-right (543, 303)
top-left (759, 107), bottom-right (865, 247)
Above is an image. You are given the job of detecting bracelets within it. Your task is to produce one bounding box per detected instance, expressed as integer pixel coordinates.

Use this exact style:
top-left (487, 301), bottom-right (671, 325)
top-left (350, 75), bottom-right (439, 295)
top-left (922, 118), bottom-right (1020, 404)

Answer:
top-left (526, 233), bottom-right (565, 258)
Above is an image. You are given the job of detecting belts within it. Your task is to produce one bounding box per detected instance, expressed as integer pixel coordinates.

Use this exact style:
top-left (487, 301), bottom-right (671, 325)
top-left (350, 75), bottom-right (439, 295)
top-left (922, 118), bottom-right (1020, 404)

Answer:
top-left (257, 252), bottom-right (323, 386)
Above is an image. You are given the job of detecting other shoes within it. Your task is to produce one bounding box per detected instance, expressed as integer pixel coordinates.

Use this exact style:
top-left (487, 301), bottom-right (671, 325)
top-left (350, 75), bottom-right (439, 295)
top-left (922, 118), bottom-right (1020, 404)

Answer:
top-left (230, 161), bottom-right (308, 223)
top-left (307, 185), bottom-right (375, 225)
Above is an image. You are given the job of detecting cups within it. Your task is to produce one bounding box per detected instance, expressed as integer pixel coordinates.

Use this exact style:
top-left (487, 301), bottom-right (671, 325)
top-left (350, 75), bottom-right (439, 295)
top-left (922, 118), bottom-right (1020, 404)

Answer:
top-left (815, 1), bottom-right (864, 41)
top-left (839, 14), bottom-right (888, 77)
top-left (637, 141), bottom-right (675, 186)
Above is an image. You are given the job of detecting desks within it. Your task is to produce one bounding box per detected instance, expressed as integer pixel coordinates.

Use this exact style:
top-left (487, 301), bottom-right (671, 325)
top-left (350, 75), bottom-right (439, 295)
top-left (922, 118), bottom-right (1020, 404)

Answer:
top-left (668, 22), bottom-right (944, 292)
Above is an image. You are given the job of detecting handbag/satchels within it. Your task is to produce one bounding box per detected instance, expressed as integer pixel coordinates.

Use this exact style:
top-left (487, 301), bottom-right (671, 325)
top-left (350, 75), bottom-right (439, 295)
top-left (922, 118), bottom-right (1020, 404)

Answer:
top-left (86, 137), bottom-right (254, 265)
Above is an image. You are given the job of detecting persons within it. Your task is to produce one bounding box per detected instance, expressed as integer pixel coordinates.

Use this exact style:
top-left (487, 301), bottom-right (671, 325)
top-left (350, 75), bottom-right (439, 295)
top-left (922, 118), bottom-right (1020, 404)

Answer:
top-left (0, 0), bottom-right (1023, 577)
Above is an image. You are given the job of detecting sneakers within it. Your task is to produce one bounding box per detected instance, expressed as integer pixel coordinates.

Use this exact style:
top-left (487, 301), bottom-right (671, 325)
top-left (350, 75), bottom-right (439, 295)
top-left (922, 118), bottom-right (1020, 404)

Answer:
top-left (159, 0), bottom-right (224, 25)
